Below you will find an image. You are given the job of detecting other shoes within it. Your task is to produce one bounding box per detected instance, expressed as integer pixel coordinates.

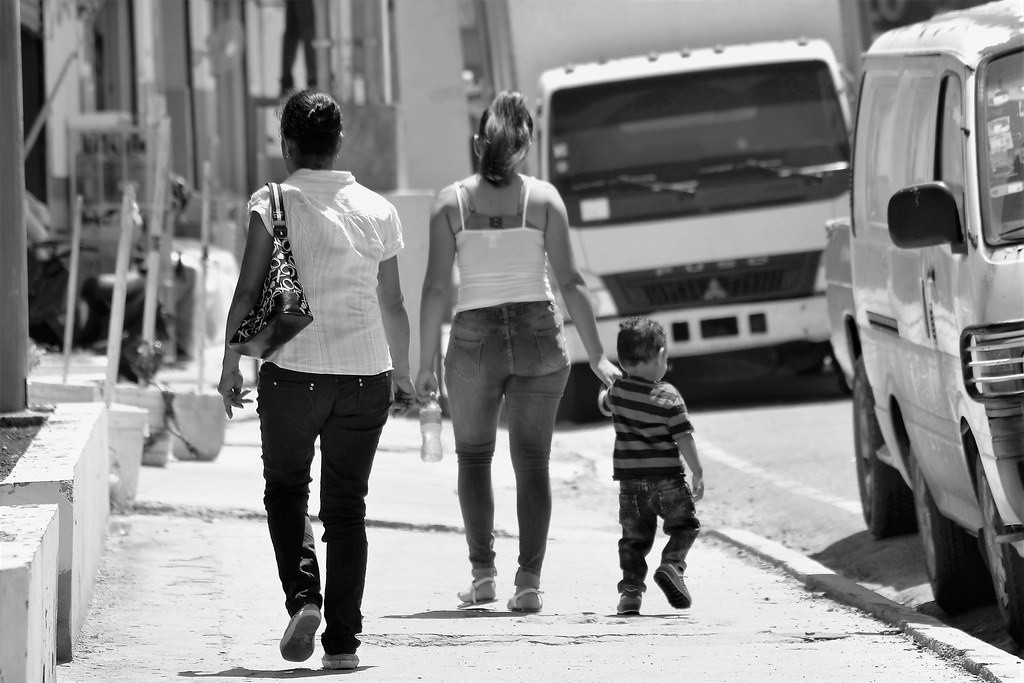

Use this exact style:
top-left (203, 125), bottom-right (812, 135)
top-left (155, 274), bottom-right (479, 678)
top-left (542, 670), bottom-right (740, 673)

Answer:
top-left (280, 603), bottom-right (321, 662)
top-left (321, 653), bottom-right (360, 669)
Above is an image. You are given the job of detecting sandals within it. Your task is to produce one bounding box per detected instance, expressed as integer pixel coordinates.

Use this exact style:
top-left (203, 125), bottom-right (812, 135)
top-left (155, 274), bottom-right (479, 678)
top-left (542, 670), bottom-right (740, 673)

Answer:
top-left (458, 576), bottom-right (496, 603)
top-left (507, 586), bottom-right (543, 611)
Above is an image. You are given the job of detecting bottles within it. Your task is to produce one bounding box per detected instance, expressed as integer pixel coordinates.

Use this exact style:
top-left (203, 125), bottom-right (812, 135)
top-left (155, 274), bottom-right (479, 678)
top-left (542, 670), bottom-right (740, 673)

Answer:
top-left (419, 392), bottom-right (443, 460)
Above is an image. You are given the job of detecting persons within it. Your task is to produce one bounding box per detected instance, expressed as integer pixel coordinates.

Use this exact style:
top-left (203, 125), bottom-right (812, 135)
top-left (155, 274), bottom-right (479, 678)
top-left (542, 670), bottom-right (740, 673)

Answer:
top-left (415, 91), bottom-right (623, 613)
top-left (597, 316), bottom-right (704, 614)
top-left (218, 88), bottom-right (415, 670)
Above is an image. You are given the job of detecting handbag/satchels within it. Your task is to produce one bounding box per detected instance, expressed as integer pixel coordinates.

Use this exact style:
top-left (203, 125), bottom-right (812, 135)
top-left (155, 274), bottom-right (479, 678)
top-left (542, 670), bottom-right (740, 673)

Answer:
top-left (228, 182), bottom-right (313, 359)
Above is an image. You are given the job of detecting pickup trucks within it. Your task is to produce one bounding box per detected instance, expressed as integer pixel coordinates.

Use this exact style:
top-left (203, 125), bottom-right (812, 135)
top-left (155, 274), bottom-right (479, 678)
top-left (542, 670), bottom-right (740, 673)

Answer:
top-left (535, 33), bottom-right (853, 423)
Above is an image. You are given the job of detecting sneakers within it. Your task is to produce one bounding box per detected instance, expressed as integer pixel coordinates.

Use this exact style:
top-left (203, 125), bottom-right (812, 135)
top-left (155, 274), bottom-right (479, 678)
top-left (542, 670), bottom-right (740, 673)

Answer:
top-left (653, 563), bottom-right (691, 609)
top-left (617, 589), bottom-right (642, 614)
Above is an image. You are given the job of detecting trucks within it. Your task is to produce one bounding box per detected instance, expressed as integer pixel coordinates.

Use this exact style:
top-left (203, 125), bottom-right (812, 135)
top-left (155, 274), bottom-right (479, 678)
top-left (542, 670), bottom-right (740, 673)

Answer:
top-left (823, 0), bottom-right (1024, 650)
